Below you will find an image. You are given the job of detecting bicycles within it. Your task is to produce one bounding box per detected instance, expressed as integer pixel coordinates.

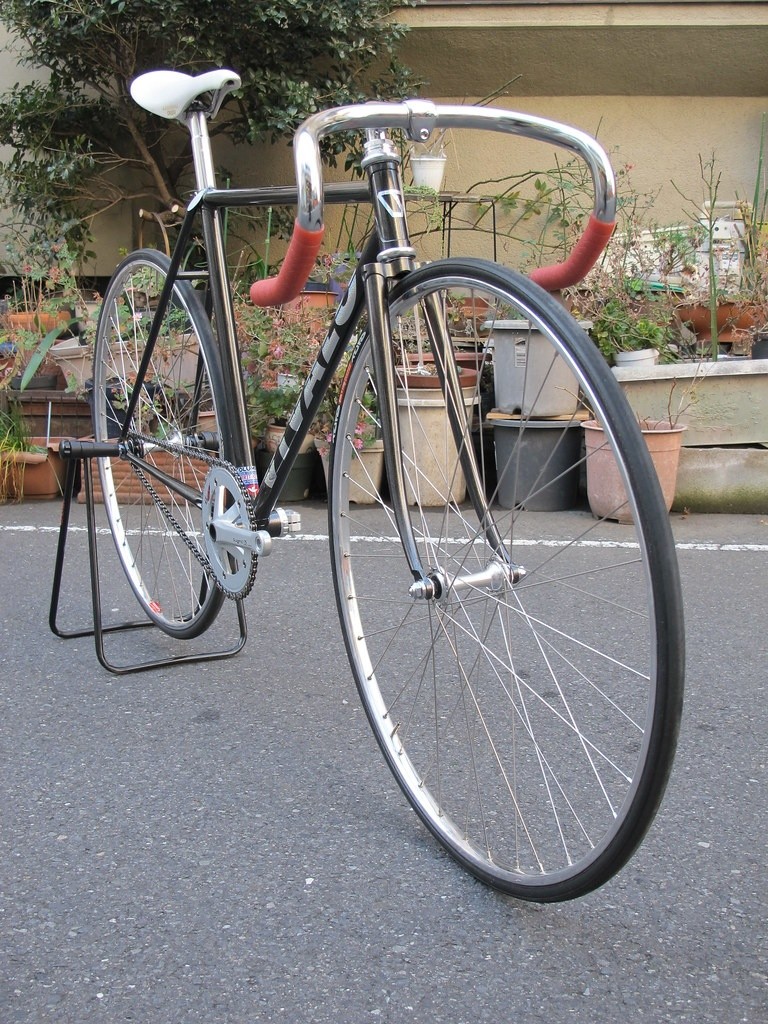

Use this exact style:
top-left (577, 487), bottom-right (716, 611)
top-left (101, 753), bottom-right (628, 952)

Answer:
top-left (48, 62), bottom-right (687, 909)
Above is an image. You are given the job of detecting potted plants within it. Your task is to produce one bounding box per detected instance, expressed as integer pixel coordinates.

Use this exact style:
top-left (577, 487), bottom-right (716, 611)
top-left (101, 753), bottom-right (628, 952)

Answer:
top-left (1, 157), bottom-right (767, 525)
